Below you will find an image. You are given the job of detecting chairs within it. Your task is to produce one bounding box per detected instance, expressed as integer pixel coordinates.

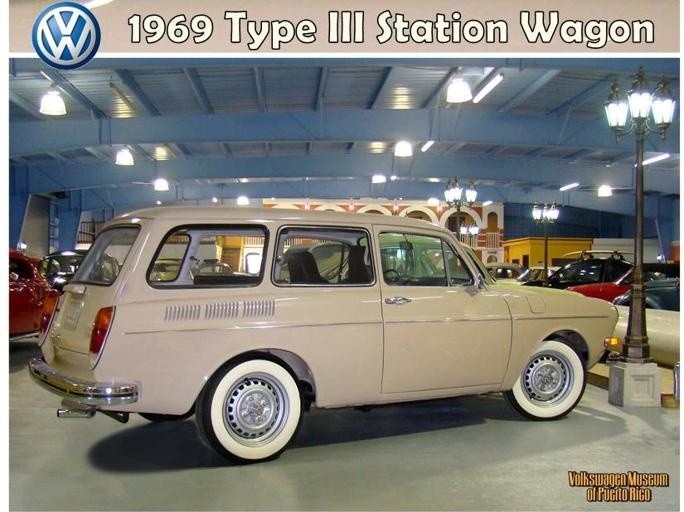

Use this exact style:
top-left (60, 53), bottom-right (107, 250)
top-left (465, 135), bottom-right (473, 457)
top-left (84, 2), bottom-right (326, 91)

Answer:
top-left (347, 245), bottom-right (374, 284)
top-left (288, 252), bottom-right (331, 284)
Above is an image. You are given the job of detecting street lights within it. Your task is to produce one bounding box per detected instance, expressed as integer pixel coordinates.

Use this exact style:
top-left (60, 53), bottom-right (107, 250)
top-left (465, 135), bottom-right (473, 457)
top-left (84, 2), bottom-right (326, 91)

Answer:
top-left (444, 174), bottom-right (477, 241)
top-left (532, 201), bottom-right (564, 279)
top-left (604, 64), bottom-right (677, 409)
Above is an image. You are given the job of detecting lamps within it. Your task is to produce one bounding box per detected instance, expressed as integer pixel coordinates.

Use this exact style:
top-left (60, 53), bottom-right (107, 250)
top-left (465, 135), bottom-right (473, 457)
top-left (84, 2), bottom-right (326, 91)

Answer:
top-left (37, 68), bottom-right (170, 192)
top-left (372, 68), bottom-right (506, 185)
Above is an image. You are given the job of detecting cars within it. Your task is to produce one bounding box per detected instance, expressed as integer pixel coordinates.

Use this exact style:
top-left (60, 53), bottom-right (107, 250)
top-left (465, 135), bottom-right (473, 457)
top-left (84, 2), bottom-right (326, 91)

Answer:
top-left (482, 251), bottom-right (680, 367)
top-left (9, 248), bottom-right (121, 342)
